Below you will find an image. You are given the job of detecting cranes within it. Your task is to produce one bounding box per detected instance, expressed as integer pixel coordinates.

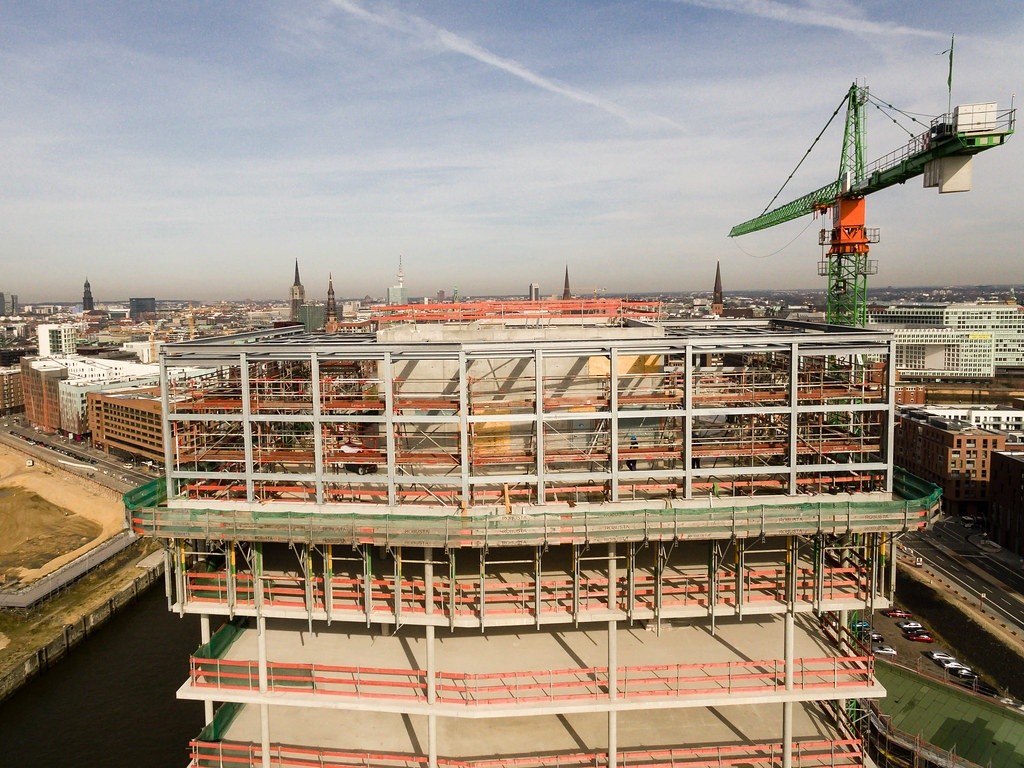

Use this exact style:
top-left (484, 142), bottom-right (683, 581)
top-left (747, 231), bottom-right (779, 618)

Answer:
top-left (729, 35), bottom-right (1019, 327)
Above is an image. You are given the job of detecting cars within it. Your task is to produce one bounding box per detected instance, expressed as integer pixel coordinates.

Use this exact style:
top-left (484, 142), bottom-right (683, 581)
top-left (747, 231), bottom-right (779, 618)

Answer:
top-left (855, 607), bottom-right (999, 694)
